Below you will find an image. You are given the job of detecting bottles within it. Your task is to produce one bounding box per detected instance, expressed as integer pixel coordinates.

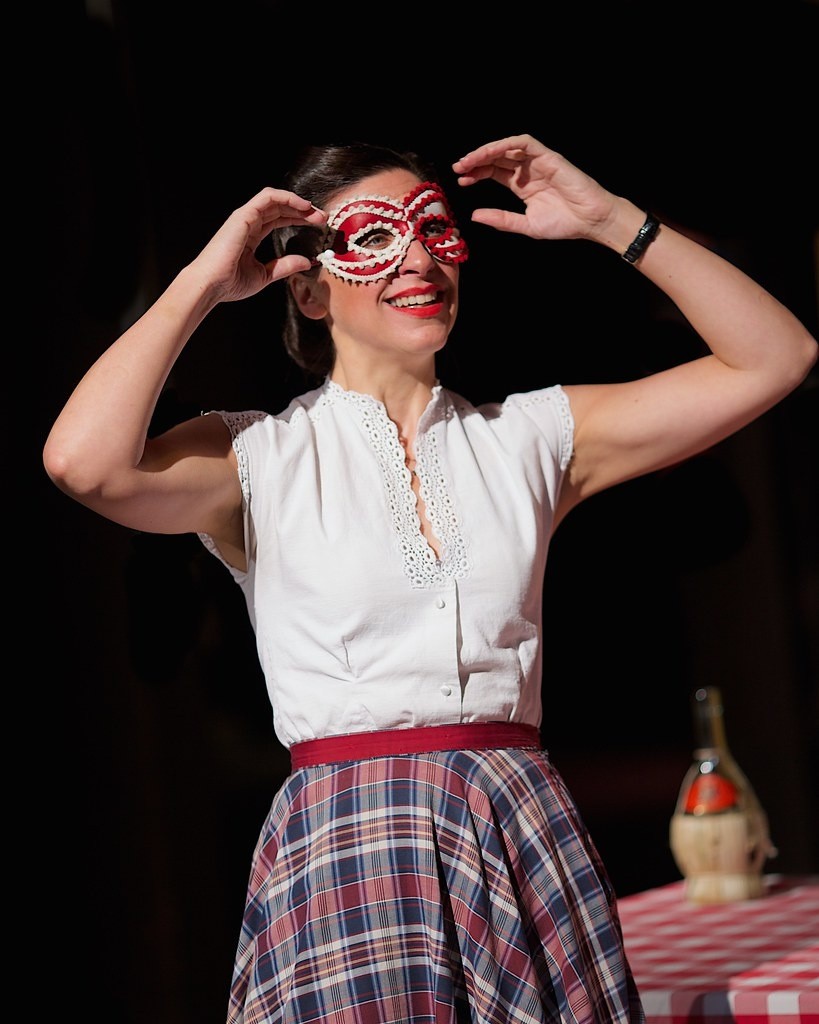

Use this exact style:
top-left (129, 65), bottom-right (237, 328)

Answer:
top-left (671, 687), bottom-right (780, 903)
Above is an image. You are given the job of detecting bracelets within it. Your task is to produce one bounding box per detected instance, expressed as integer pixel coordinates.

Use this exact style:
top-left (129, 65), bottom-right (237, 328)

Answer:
top-left (621, 212), bottom-right (662, 264)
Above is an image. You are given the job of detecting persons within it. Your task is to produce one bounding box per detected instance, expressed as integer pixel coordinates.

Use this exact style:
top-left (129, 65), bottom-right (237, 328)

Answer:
top-left (43, 134), bottom-right (819, 1024)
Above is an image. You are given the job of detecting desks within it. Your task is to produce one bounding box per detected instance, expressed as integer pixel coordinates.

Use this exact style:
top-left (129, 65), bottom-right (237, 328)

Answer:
top-left (614, 873), bottom-right (819, 1024)
